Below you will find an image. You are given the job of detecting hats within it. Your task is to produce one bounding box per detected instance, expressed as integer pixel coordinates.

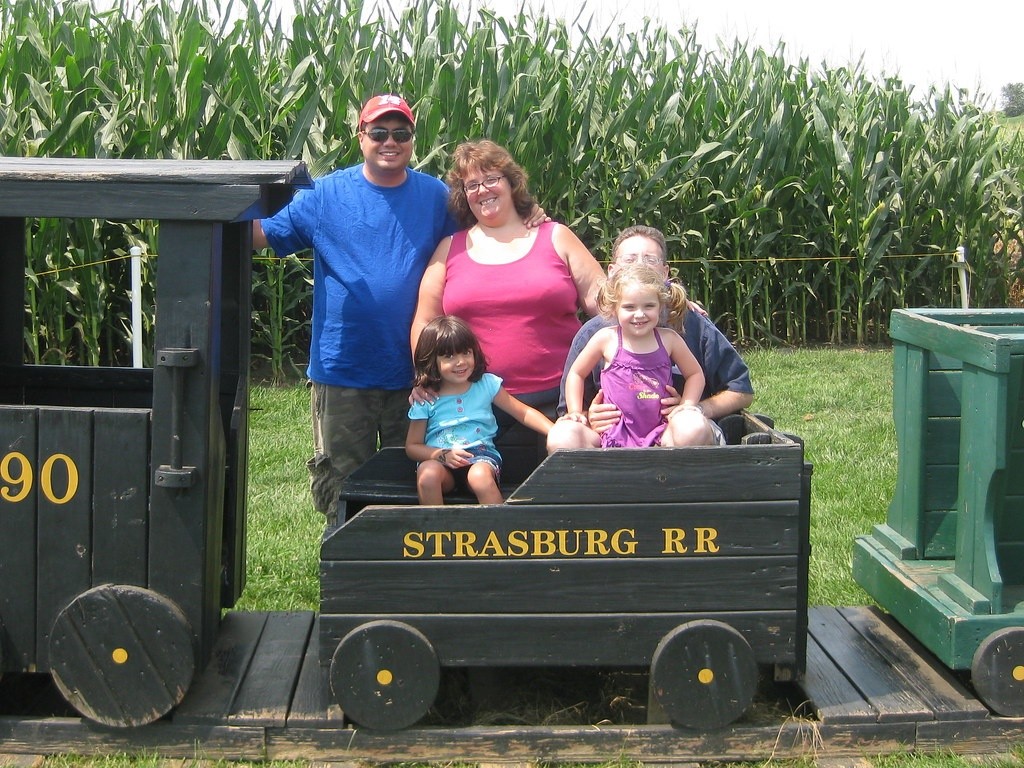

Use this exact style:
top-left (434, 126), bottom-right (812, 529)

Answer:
top-left (359, 96), bottom-right (415, 132)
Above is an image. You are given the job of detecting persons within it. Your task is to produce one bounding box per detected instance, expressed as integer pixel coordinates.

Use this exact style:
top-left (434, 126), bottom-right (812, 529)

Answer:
top-left (404, 315), bottom-right (555, 506)
top-left (546, 224), bottom-right (755, 448)
top-left (556, 259), bottom-right (706, 448)
top-left (251, 94), bottom-right (551, 528)
top-left (409, 140), bottom-right (608, 424)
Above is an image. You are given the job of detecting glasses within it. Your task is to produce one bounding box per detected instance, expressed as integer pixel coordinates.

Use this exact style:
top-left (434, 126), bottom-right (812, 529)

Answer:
top-left (364, 127), bottom-right (413, 143)
top-left (615, 252), bottom-right (666, 267)
top-left (464, 175), bottom-right (506, 193)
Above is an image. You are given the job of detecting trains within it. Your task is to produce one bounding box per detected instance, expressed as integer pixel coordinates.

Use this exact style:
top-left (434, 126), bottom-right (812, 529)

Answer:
top-left (0, 158), bottom-right (1024, 729)
